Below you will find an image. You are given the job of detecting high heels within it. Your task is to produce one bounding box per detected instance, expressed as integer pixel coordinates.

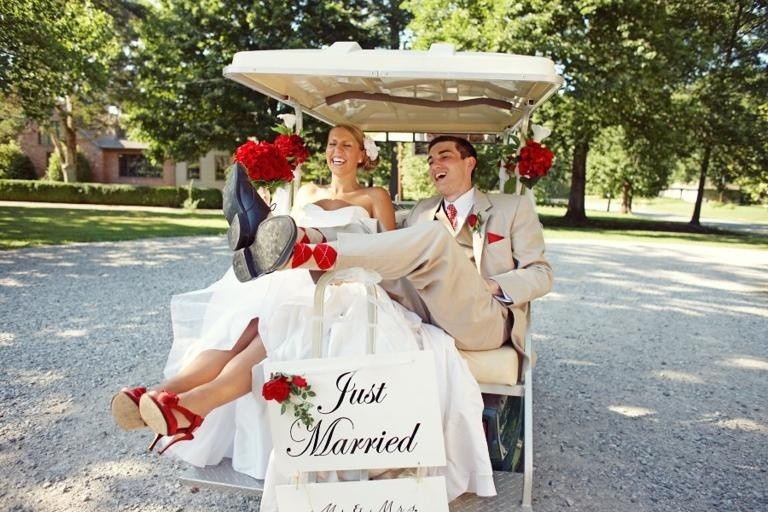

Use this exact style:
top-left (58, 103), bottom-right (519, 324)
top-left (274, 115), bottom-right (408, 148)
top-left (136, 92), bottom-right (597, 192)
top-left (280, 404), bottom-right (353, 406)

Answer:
top-left (138, 389), bottom-right (205, 456)
top-left (109, 385), bottom-right (166, 452)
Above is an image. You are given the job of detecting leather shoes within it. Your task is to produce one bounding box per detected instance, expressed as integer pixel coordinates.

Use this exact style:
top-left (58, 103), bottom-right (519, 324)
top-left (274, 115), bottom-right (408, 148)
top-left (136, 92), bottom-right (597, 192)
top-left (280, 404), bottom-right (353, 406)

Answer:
top-left (222, 161), bottom-right (272, 252)
top-left (233, 213), bottom-right (299, 282)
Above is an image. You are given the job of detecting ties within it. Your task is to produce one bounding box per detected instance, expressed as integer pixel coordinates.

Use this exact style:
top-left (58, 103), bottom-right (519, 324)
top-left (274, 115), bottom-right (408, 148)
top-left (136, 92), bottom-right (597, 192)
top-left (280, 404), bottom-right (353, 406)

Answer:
top-left (445, 204), bottom-right (457, 230)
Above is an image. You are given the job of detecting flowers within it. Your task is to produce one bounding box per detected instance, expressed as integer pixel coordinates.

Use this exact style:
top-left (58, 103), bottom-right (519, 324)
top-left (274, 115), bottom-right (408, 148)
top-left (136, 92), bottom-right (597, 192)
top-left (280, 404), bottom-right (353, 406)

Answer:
top-left (493, 132), bottom-right (555, 194)
top-left (261, 369), bottom-right (319, 429)
top-left (467, 211), bottom-right (483, 237)
top-left (222, 120), bottom-right (311, 206)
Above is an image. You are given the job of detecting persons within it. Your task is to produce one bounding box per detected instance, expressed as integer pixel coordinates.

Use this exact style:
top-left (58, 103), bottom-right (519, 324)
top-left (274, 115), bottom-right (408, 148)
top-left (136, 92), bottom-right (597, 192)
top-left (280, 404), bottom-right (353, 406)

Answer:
top-left (224, 135), bottom-right (552, 351)
top-left (111, 124), bottom-right (395, 454)
top-left (623, 181), bottom-right (633, 213)
